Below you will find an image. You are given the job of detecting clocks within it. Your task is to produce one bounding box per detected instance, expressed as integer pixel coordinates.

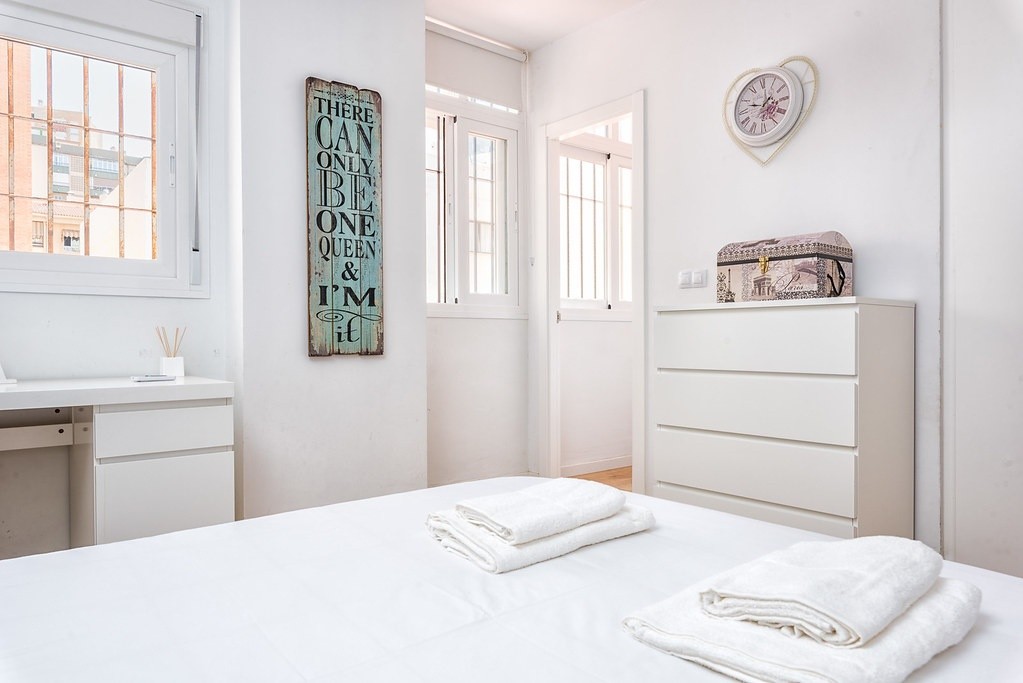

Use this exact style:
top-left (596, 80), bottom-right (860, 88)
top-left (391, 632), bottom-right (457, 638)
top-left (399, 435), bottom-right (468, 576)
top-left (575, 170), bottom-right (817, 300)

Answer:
top-left (722, 55), bottom-right (820, 169)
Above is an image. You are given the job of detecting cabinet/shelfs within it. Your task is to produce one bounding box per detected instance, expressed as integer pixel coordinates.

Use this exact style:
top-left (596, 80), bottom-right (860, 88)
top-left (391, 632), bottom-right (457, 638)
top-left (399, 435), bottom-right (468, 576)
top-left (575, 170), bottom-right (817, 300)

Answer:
top-left (66, 398), bottom-right (236, 550)
top-left (653, 296), bottom-right (916, 540)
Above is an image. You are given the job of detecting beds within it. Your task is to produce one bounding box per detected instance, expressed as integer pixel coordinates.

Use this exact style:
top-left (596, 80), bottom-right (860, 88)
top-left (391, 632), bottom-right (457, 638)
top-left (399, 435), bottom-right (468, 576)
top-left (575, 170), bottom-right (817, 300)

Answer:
top-left (0, 475), bottom-right (1023, 682)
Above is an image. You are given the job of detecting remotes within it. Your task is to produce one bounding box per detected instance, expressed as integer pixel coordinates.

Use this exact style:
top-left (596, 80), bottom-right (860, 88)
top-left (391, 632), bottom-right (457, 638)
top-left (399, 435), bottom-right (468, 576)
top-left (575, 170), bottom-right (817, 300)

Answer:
top-left (131, 374), bottom-right (176, 381)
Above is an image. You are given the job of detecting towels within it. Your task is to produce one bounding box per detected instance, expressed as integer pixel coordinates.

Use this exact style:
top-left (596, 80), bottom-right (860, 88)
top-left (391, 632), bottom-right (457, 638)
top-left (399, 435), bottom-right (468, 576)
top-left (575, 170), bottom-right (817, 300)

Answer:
top-left (423, 477), bottom-right (655, 576)
top-left (618, 533), bottom-right (982, 683)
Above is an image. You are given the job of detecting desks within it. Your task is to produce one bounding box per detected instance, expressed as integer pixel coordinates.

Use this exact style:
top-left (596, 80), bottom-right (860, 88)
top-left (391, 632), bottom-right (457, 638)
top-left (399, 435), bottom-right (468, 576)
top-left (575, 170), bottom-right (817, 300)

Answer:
top-left (0, 376), bottom-right (236, 411)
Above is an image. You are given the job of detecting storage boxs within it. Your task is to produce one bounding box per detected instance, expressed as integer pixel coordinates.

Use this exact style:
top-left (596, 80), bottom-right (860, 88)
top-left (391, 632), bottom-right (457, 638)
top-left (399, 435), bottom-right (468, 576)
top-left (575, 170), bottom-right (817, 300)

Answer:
top-left (716, 231), bottom-right (854, 304)
